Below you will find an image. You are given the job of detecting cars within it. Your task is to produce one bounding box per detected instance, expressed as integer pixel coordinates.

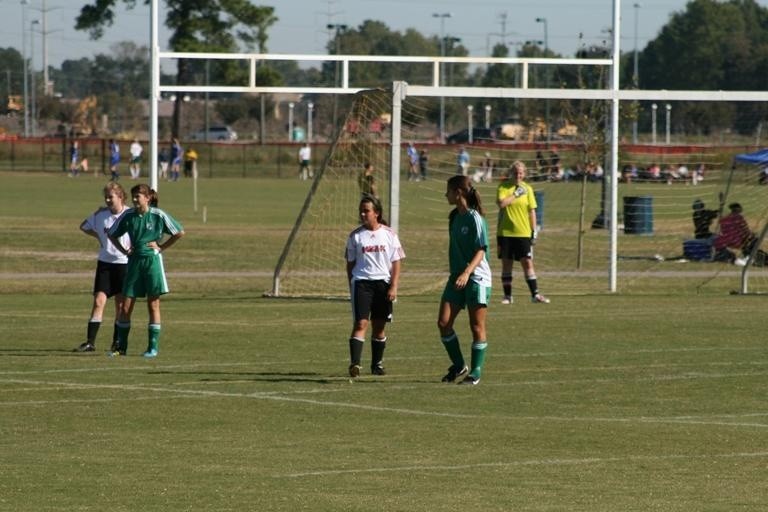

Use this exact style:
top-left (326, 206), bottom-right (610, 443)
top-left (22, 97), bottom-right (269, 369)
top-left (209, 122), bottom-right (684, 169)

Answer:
top-left (446, 127), bottom-right (497, 143)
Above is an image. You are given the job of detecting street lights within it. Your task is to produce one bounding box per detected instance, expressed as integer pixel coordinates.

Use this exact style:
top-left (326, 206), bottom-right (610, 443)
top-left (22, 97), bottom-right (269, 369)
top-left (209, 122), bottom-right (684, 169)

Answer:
top-left (307, 102), bottom-right (314, 141)
top-left (631, 3), bottom-right (642, 144)
top-left (432, 12), bottom-right (452, 142)
top-left (326, 23), bottom-right (348, 143)
top-left (26, 18), bottom-right (44, 137)
top-left (20, 0), bottom-right (29, 136)
top-left (665, 104), bottom-right (672, 142)
top-left (467, 104), bottom-right (474, 143)
top-left (485, 105), bottom-right (492, 128)
top-left (536, 19), bottom-right (551, 143)
top-left (509, 41), bottom-right (523, 142)
top-left (651, 103), bottom-right (658, 143)
top-left (444, 36), bottom-right (461, 131)
top-left (287, 101), bottom-right (295, 141)
top-left (525, 39), bottom-right (543, 142)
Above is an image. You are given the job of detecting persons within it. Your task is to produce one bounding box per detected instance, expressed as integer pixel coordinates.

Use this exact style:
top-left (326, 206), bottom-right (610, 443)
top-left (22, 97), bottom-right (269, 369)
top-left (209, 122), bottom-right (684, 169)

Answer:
top-left (493, 160), bottom-right (552, 305)
top-left (296, 141), bottom-right (314, 181)
top-left (71, 180), bottom-right (131, 357)
top-left (434, 175), bottom-right (492, 385)
top-left (356, 163), bottom-right (377, 198)
top-left (406, 138), bottom-right (704, 186)
top-left (344, 194), bottom-right (405, 377)
top-left (721, 202), bottom-right (757, 254)
top-left (101, 182), bottom-right (185, 357)
top-left (58, 129), bottom-right (195, 181)
top-left (292, 121), bottom-right (306, 142)
top-left (692, 192), bottom-right (725, 239)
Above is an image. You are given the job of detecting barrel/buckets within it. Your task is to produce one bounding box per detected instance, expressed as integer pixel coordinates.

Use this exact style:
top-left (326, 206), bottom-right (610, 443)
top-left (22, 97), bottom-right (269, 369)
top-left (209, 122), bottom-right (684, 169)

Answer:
top-left (534, 190), bottom-right (544, 228)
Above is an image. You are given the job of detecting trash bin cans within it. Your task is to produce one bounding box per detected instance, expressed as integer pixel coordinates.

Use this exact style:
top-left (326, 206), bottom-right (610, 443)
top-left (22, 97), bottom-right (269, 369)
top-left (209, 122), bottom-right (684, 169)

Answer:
top-left (535, 191), bottom-right (543, 230)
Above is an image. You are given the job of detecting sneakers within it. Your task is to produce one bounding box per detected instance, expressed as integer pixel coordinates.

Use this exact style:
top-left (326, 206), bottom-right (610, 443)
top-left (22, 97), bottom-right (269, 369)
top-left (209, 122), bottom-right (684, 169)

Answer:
top-left (74, 338), bottom-right (158, 358)
top-left (460, 375), bottom-right (480, 385)
top-left (501, 297), bottom-right (511, 304)
top-left (441, 365), bottom-right (468, 382)
top-left (371, 364), bottom-right (384, 374)
top-left (531, 294), bottom-right (549, 303)
top-left (348, 364), bottom-right (360, 377)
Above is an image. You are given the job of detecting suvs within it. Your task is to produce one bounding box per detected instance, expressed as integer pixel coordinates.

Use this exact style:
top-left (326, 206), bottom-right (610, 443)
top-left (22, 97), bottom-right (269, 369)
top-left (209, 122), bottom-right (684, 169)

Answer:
top-left (188, 125), bottom-right (237, 143)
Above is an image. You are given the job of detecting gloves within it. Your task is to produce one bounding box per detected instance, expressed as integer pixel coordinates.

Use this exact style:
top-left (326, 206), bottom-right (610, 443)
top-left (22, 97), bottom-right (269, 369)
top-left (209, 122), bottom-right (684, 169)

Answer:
top-left (512, 185), bottom-right (525, 198)
top-left (529, 231), bottom-right (537, 245)
